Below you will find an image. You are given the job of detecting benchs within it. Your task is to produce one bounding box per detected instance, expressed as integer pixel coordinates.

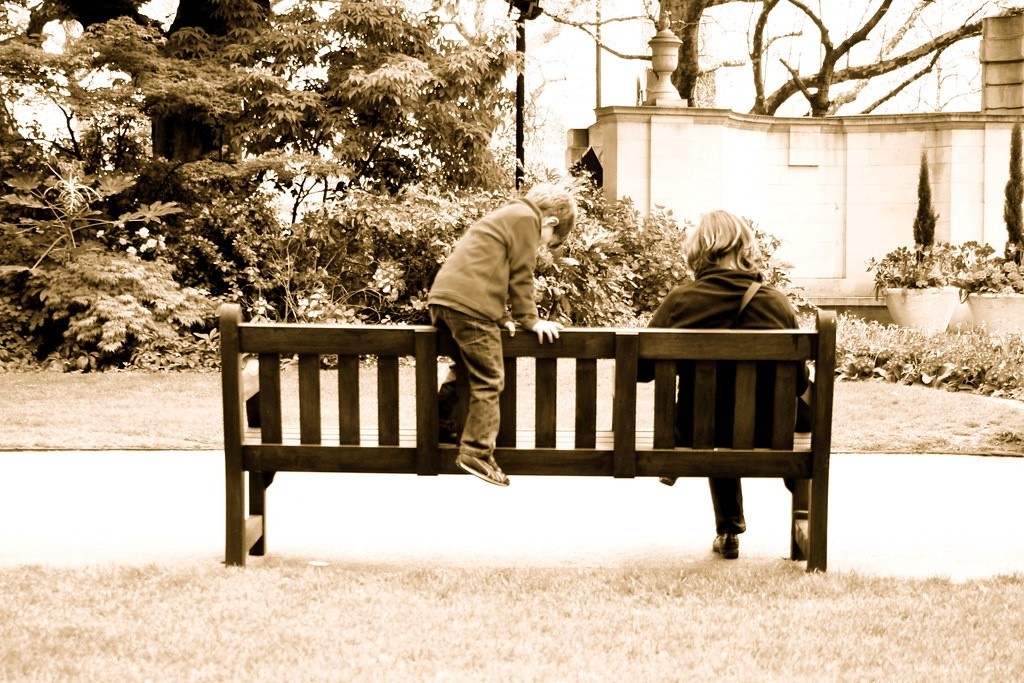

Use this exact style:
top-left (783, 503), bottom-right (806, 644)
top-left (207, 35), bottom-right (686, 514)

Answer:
top-left (214, 301), bottom-right (839, 572)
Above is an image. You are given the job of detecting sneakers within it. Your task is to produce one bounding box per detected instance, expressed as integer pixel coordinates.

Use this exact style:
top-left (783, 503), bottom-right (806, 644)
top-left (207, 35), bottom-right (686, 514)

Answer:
top-left (452, 453), bottom-right (509, 488)
top-left (437, 430), bottom-right (458, 449)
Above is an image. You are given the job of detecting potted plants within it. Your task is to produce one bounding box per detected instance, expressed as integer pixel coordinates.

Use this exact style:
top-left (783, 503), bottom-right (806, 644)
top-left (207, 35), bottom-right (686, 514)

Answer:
top-left (949, 240), bottom-right (1024, 339)
top-left (864, 241), bottom-right (964, 336)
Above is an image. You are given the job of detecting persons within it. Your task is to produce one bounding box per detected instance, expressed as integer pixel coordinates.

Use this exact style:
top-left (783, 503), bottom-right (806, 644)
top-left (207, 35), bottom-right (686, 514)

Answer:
top-left (638, 209), bottom-right (814, 560)
top-left (424, 181), bottom-right (579, 489)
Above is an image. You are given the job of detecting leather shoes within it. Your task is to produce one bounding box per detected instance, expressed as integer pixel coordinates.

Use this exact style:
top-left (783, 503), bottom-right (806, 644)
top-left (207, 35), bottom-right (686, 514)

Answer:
top-left (712, 533), bottom-right (740, 559)
top-left (660, 476), bottom-right (678, 485)
